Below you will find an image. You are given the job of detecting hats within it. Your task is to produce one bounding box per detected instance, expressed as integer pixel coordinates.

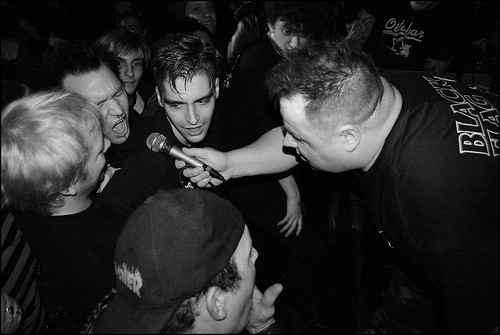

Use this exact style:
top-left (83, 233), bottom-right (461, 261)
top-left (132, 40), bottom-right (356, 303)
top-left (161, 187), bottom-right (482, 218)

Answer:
top-left (93, 188), bottom-right (245, 335)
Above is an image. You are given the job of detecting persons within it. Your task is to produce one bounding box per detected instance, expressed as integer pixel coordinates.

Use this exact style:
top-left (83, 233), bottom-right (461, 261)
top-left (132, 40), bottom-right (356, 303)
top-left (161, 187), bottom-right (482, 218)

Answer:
top-left (0, 191), bottom-right (48, 334)
top-left (128, 32), bottom-right (230, 200)
top-left (174, 37), bottom-right (499, 334)
top-left (1, 1), bottom-right (225, 109)
top-left (224, 0), bottom-right (335, 334)
top-left (336, 1), bottom-right (500, 94)
top-left (45, 40), bottom-right (146, 174)
top-left (92, 189), bottom-right (282, 335)
top-left (1, 90), bottom-right (144, 334)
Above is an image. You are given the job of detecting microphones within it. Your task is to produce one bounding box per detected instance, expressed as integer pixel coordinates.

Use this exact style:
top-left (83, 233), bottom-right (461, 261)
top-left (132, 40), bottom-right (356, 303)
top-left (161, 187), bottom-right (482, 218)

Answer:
top-left (146, 133), bottom-right (224, 181)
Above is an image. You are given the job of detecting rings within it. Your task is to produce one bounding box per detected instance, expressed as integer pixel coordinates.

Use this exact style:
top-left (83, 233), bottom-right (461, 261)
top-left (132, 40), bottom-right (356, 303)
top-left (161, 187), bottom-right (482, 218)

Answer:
top-left (5, 306), bottom-right (14, 314)
top-left (14, 311), bottom-right (20, 316)
